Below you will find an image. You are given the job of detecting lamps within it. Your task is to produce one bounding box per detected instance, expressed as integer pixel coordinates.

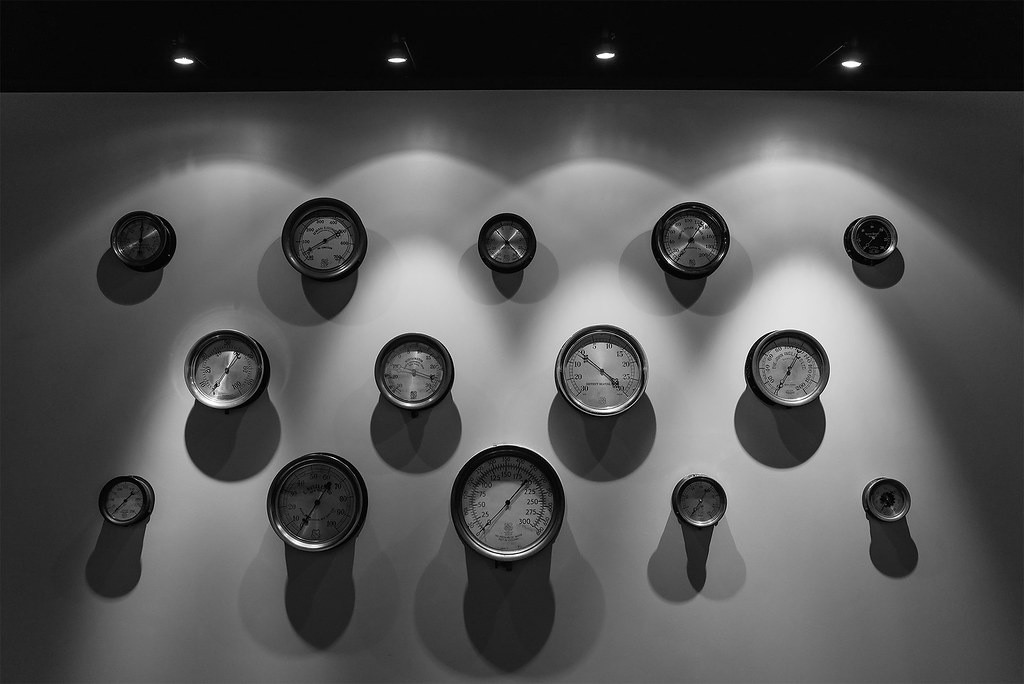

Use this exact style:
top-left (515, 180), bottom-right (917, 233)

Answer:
top-left (384, 32), bottom-right (416, 69)
top-left (168, 28), bottom-right (209, 68)
top-left (594, 26), bottom-right (619, 63)
top-left (808, 35), bottom-right (864, 73)
